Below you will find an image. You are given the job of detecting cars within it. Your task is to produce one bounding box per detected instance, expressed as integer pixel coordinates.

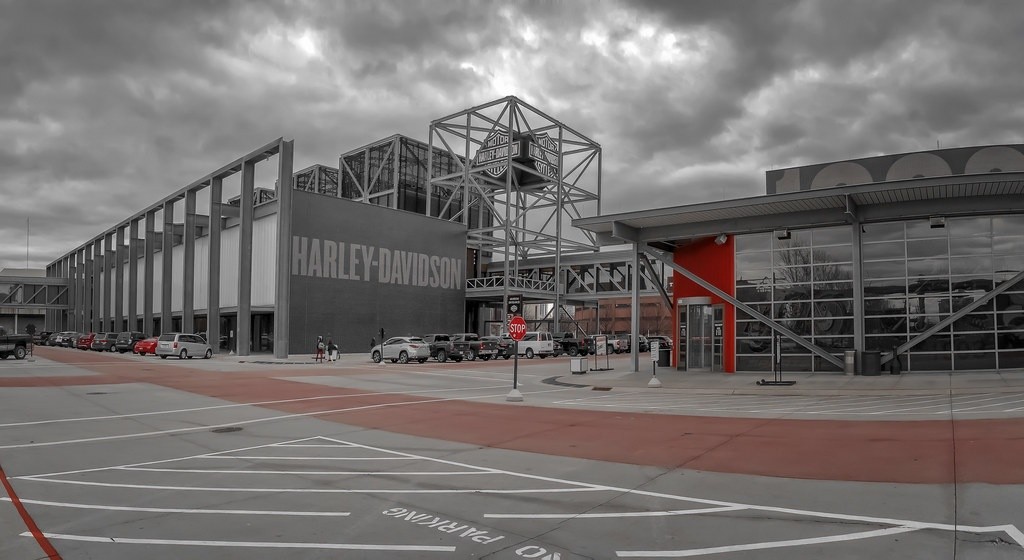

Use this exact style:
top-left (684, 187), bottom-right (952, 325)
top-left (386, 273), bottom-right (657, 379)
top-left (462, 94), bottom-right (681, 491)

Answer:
top-left (33, 331), bottom-right (87, 349)
top-left (548, 331), bottom-right (648, 357)
top-left (134, 336), bottom-right (159, 356)
top-left (370, 336), bottom-right (431, 363)
top-left (479, 335), bottom-right (515, 360)
top-left (646, 336), bottom-right (673, 350)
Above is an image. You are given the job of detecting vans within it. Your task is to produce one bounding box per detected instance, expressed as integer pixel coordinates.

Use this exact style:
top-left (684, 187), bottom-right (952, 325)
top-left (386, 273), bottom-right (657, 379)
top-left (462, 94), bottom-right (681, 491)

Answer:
top-left (501, 332), bottom-right (554, 360)
top-left (155, 333), bottom-right (213, 359)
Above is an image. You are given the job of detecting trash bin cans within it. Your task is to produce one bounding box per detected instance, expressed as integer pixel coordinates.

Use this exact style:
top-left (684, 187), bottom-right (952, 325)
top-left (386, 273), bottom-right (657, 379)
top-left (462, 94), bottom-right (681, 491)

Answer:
top-left (844, 350), bottom-right (856, 374)
top-left (658, 348), bottom-right (671, 367)
top-left (862, 350), bottom-right (881, 376)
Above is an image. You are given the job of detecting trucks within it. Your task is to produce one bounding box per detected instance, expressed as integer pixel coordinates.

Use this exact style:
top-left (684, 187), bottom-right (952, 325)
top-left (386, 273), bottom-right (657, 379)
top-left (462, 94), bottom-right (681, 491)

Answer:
top-left (0, 333), bottom-right (34, 360)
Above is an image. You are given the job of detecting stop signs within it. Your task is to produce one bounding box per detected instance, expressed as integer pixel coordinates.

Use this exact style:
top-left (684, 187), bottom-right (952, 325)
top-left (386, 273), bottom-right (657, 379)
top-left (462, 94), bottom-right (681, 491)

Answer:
top-left (509, 317), bottom-right (527, 342)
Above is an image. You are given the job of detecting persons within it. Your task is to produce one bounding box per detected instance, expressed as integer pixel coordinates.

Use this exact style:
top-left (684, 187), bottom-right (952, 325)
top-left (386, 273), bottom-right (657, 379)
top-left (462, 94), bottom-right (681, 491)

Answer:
top-left (371, 336), bottom-right (376, 347)
top-left (327, 339), bottom-right (338, 364)
top-left (316, 336), bottom-right (323, 363)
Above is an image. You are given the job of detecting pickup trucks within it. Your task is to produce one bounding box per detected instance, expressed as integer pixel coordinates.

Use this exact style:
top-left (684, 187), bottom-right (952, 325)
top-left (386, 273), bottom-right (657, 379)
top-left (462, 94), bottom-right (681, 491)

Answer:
top-left (452, 333), bottom-right (498, 360)
top-left (422, 333), bottom-right (471, 362)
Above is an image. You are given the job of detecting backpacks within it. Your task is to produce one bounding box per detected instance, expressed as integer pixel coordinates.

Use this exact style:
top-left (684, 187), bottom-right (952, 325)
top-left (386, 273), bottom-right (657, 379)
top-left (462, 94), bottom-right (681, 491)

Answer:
top-left (318, 342), bottom-right (324, 349)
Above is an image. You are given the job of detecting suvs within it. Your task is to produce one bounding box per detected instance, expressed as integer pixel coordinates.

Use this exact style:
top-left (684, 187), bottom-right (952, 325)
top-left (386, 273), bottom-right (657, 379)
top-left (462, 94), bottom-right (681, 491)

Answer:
top-left (78, 333), bottom-right (97, 350)
top-left (115, 331), bottom-right (151, 354)
top-left (91, 332), bottom-right (121, 351)
